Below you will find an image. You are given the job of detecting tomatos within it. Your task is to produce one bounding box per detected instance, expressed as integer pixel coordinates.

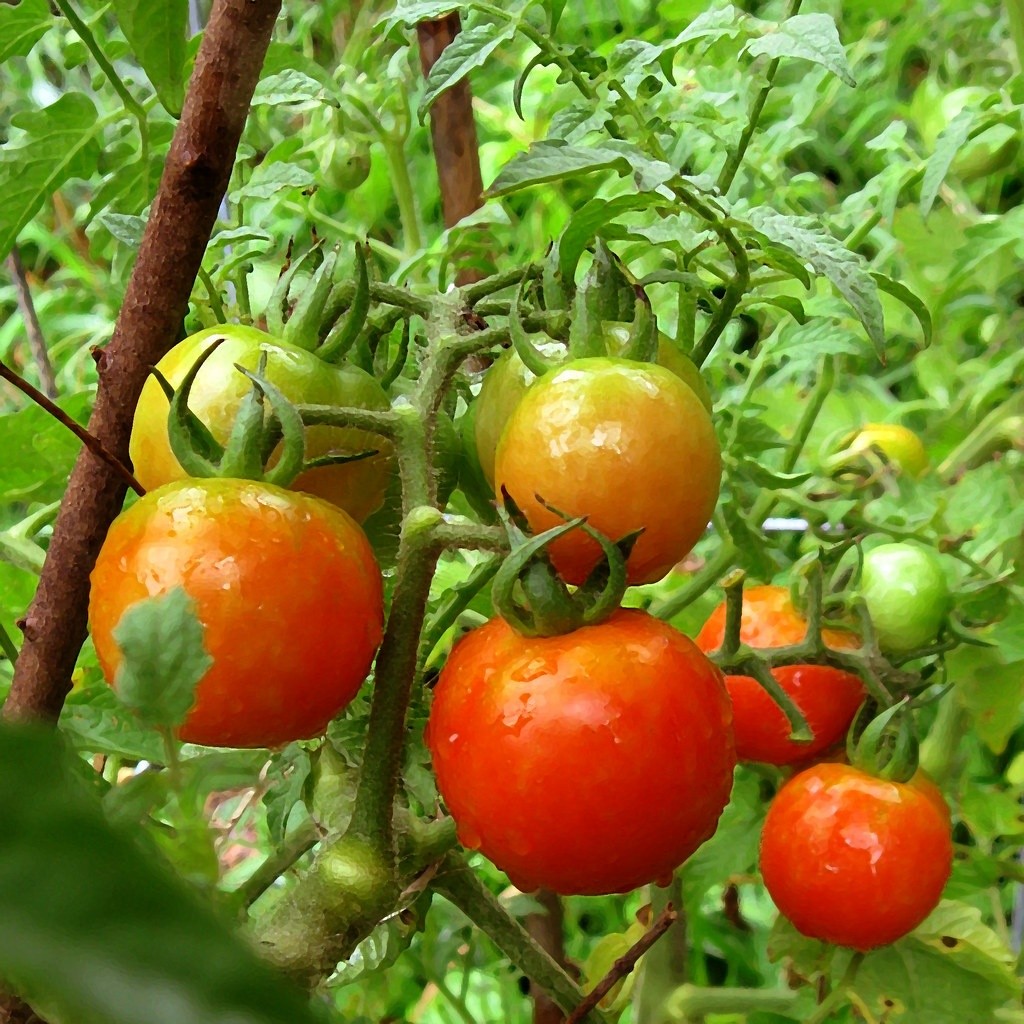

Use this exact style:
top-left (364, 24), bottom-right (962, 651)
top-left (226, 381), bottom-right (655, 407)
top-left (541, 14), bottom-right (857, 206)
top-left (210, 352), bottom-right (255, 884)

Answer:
top-left (89, 322), bottom-right (952, 950)
